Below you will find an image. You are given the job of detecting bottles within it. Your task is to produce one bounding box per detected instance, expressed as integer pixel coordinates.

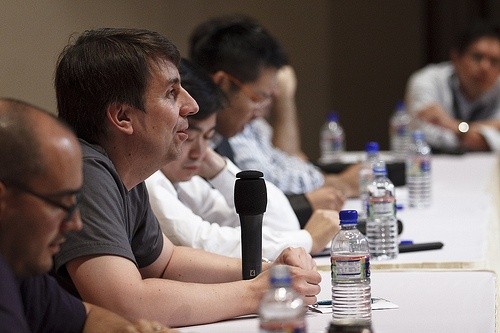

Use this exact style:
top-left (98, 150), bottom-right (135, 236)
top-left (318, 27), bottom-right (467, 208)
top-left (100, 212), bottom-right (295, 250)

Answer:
top-left (330, 210), bottom-right (372, 333)
top-left (359, 143), bottom-right (381, 220)
top-left (389, 105), bottom-right (414, 162)
top-left (405, 132), bottom-right (432, 209)
top-left (319, 113), bottom-right (345, 164)
top-left (259, 264), bottom-right (306, 333)
top-left (364, 162), bottom-right (399, 260)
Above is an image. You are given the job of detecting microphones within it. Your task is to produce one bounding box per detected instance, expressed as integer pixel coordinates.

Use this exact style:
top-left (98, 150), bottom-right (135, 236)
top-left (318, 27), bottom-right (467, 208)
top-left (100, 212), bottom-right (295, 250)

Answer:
top-left (233, 170), bottom-right (267, 280)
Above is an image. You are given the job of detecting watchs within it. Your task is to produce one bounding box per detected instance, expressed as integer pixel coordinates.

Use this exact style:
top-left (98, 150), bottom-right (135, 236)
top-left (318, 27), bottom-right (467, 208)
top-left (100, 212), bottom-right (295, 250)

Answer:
top-left (454, 119), bottom-right (474, 137)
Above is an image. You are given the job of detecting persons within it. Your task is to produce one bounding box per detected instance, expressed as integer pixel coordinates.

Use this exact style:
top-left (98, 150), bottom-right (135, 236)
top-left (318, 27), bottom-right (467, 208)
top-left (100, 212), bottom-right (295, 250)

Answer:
top-left (145, 15), bottom-right (370, 264)
top-left (49, 27), bottom-right (322, 328)
top-left (0, 97), bottom-right (177, 333)
top-left (403, 31), bottom-right (500, 154)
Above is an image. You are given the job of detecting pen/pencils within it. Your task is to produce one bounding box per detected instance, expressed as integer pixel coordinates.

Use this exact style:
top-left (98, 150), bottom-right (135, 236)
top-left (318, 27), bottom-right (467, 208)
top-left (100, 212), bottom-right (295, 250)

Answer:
top-left (315, 298), bottom-right (374, 305)
top-left (307, 304), bottom-right (323, 313)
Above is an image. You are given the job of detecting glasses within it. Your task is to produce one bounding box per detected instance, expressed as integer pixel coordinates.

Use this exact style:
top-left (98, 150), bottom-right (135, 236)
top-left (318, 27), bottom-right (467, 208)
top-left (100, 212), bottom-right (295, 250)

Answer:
top-left (223, 70), bottom-right (275, 114)
top-left (0, 175), bottom-right (83, 223)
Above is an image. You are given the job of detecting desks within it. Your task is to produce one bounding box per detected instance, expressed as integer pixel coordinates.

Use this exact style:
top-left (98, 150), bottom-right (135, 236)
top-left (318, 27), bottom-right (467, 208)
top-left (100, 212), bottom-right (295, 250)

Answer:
top-left (174, 152), bottom-right (499, 333)
